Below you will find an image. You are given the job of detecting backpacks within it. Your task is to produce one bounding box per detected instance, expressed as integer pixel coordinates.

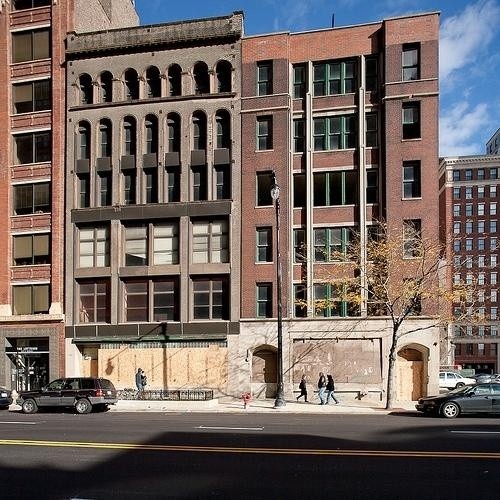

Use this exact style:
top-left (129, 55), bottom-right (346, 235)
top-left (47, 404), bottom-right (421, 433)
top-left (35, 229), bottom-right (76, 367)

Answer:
top-left (298, 382), bottom-right (303, 389)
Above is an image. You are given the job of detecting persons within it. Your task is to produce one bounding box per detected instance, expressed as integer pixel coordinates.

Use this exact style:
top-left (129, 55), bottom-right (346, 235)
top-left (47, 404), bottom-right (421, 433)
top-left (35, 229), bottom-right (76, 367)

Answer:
top-left (325, 375), bottom-right (339, 405)
top-left (317, 372), bottom-right (327, 405)
top-left (134, 368), bottom-right (144, 400)
top-left (141, 371), bottom-right (148, 386)
top-left (296, 374), bottom-right (309, 403)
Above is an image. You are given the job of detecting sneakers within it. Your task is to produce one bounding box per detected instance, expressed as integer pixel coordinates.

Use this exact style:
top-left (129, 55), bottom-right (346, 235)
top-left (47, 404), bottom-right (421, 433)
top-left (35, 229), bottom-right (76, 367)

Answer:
top-left (296, 397), bottom-right (340, 405)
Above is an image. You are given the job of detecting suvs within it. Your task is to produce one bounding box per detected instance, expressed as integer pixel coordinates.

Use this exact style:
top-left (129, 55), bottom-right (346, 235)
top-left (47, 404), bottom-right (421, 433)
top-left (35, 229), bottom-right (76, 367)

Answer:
top-left (17, 377), bottom-right (118, 414)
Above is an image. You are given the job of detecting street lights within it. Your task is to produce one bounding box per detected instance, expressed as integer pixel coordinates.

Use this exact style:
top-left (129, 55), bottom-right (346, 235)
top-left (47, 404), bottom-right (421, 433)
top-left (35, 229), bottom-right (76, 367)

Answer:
top-left (269, 169), bottom-right (285, 410)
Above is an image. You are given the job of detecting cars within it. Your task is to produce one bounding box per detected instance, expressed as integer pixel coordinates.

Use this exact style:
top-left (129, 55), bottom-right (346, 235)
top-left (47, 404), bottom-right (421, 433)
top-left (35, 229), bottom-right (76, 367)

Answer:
top-left (415, 383), bottom-right (500, 419)
top-left (471, 374), bottom-right (500, 384)
top-left (439, 372), bottom-right (476, 391)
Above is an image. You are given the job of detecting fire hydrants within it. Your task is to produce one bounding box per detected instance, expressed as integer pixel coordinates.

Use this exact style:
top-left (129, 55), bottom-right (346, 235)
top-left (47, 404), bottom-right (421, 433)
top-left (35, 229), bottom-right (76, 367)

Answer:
top-left (241, 393), bottom-right (251, 409)
